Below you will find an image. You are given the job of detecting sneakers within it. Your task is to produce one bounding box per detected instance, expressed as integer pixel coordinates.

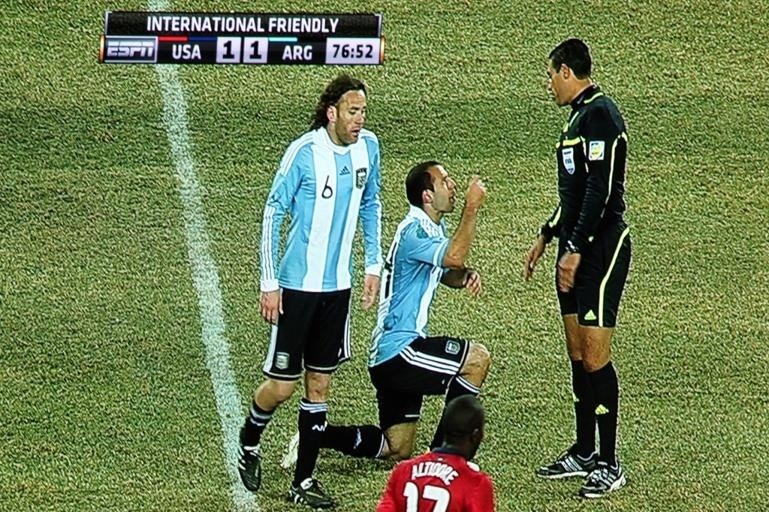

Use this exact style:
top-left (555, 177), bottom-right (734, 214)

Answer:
top-left (285, 476), bottom-right (335, 510)
top-left (534, 450), bottom-right (600, 479)
top-left (238, 426), bottom-right (263, 493)
top-left (578, 457), bottom-right (628, 499)
top-left (281, 429), bottom-right (302, 470)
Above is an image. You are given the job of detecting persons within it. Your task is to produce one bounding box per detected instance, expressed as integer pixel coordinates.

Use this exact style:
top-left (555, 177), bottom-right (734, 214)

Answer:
top-left (518, 37), bottom-right (633, 497)
top-left (372, 390), bottom-right (496, 512)
top-left (231, 73), bottom-right (384, 510)
top-left (272, 158), bottom-right (496, 478)
